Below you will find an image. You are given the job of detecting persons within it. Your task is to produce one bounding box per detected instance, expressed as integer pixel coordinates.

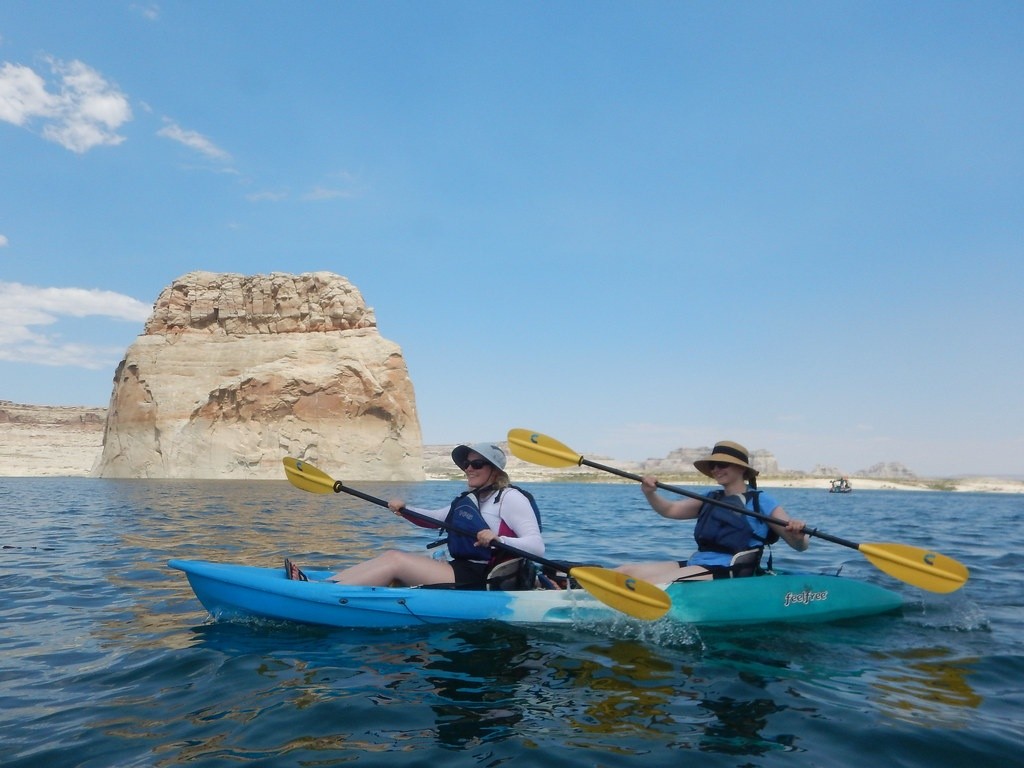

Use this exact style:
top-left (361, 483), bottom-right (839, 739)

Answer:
top-left (612, 441), bottom-right (809, 585)
top-left (285, 442), bottom-right (545, 590)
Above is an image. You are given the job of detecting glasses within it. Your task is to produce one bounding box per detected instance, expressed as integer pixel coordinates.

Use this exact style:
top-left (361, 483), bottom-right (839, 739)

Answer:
top-left (710, 462), bottom-right (734, 470)
top-left (462, 458), bottom-right (488, 469)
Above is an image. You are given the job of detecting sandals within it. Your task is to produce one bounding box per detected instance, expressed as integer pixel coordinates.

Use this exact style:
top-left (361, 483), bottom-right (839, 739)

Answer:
top-left (537, 573), bottom-right (563, 590)
top-left (285, 557), bottom-right (308, 583)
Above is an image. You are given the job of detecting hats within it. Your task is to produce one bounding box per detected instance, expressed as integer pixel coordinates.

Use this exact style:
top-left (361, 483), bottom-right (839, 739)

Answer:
top-left (452, 442), bottom-right (507, 475)
top-left (694, 441), bottom-right (759, 480)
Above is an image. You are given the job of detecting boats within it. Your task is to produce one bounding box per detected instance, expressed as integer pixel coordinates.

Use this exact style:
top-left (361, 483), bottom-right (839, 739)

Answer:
top-left (166, 556), bottom-right (912, 634)
top-left (830, 475), bottom-right (853, 494)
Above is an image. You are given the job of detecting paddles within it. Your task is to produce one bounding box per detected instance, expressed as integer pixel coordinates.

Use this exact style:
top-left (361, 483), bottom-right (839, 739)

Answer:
top-left (505, 427), bottom-right (970, 593)
top-left (281, 454), bottom-right (671, 619)
